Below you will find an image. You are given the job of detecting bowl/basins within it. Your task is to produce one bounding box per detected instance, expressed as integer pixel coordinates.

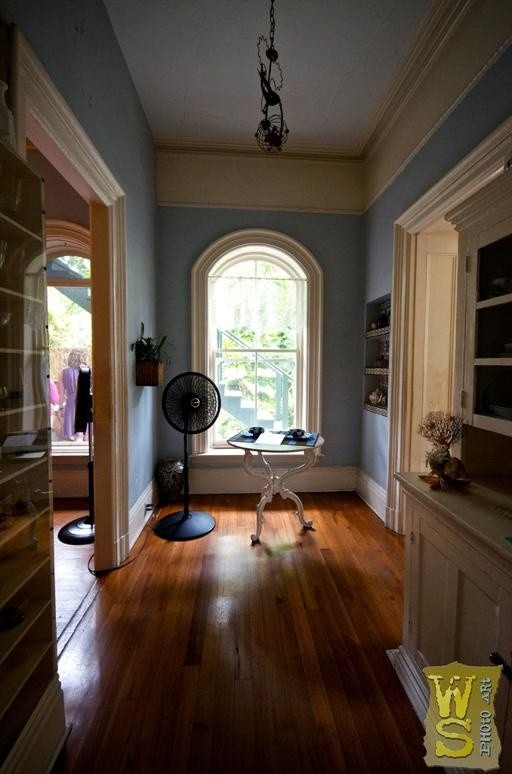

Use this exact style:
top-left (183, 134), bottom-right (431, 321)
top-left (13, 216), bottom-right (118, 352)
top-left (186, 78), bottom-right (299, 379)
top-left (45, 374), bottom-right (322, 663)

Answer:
top-left (249, 427), bottom-right (264, 433)
top-left (289, 429), bottom-right (304, 436)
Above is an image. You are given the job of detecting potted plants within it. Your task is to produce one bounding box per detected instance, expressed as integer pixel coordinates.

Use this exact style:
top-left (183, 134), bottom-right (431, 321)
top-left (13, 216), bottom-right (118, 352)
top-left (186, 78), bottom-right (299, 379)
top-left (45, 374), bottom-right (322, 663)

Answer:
top-left (130, 322), bottom-right (175, 387)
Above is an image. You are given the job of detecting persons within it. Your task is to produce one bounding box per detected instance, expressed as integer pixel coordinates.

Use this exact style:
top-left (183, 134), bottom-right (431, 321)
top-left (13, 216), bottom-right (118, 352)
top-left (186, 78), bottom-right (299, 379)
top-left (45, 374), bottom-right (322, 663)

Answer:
top-left (55, 345), bottom-right (92, 443)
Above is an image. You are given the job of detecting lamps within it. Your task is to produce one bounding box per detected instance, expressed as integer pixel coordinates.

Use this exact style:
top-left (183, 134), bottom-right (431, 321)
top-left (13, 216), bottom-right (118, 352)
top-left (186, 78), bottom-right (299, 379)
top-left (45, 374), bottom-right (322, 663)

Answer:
top-left (254, 0), bottom-right (290, 155)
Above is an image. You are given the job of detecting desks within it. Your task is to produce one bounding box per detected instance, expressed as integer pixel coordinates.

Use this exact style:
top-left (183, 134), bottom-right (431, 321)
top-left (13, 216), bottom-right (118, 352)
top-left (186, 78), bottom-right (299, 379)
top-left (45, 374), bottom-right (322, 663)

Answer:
top-left (226, 429), bottom-right (325, 544)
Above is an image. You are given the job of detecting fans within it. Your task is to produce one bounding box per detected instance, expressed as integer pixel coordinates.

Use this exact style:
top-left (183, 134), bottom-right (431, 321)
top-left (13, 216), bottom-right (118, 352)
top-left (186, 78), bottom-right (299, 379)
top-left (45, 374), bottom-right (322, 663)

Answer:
top-left (57, 367), bottom-right (95, 546)
top-left (153, 370), bottom-right (221, 542)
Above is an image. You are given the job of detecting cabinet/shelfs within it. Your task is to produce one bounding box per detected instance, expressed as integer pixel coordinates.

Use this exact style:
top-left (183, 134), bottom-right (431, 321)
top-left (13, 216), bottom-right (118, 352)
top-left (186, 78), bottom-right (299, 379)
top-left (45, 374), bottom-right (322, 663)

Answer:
top-left (0, 135), bottom-right (74, 773)
